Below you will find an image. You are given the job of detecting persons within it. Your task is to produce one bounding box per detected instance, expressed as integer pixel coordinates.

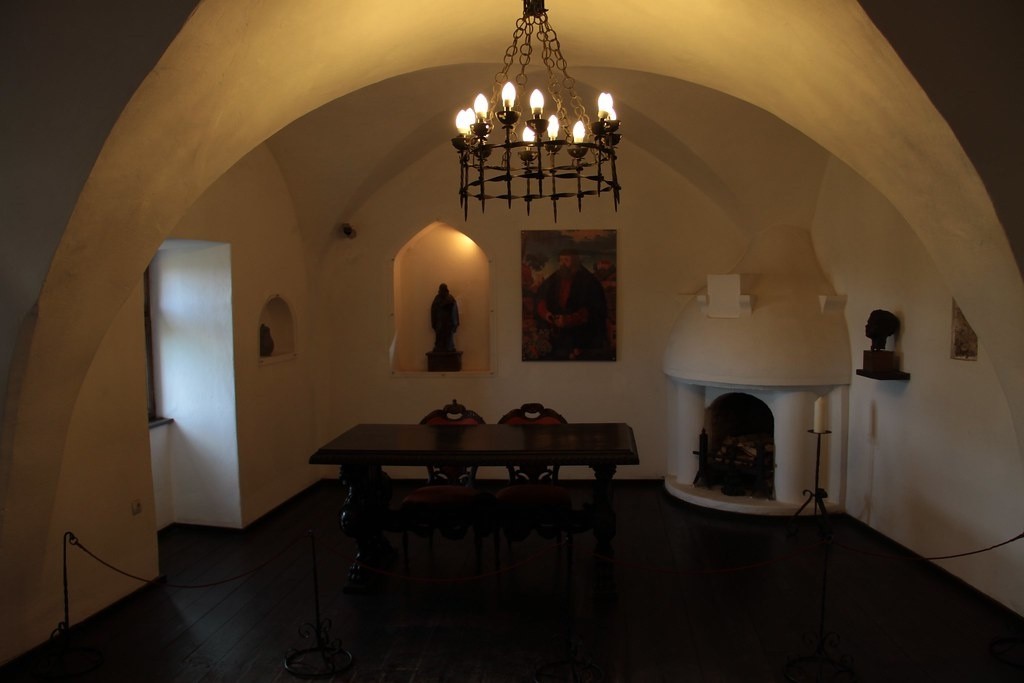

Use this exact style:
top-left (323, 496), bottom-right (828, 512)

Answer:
top-left (431, 284), bottom-right (460, 352)
top-left (866, 310), bottom-right (899, 349)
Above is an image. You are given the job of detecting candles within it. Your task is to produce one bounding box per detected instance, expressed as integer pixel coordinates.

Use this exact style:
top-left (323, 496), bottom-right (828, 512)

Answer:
top-left (813, 395), bottom-right (826, 433)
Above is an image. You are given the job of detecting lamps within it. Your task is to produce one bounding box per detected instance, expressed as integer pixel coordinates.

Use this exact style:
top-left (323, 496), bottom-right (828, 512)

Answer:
top-left (452, 0), bottom-right (624, 225)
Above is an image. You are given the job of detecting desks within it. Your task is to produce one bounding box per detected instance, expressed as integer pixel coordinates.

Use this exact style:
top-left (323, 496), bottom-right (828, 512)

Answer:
top-left (308, 423), bottom-right (640, 601)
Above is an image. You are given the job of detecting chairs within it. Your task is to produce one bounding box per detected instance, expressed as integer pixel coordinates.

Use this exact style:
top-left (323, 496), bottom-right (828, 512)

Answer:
top-left (488, 403), bottom-right (574, 584)
top-left (399, 400), bottom-right (488, 584)
